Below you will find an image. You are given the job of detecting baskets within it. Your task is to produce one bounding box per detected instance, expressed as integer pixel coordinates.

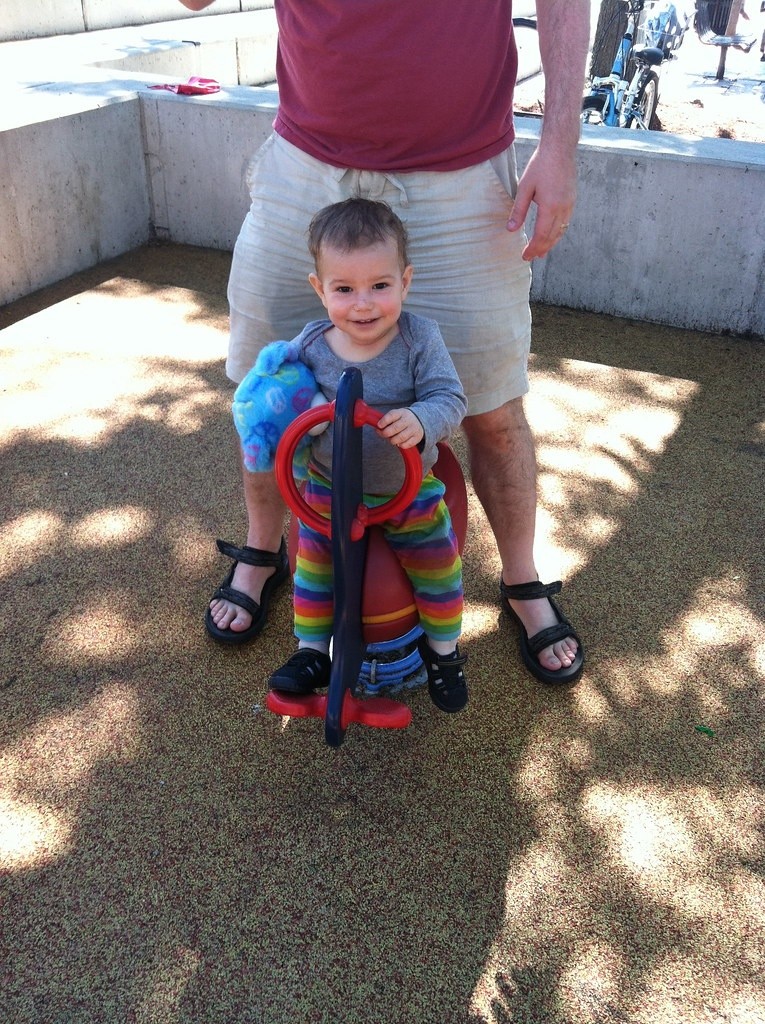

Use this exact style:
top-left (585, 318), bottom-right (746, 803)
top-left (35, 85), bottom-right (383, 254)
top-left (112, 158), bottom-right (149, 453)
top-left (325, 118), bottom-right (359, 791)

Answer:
top-left (637, 20), bottom-right (689, 60)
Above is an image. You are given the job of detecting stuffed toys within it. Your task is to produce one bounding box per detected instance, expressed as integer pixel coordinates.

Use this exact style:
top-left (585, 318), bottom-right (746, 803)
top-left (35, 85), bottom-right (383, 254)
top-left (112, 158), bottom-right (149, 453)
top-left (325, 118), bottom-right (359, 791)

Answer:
top-left (231, 340), bottom-right (331, 482)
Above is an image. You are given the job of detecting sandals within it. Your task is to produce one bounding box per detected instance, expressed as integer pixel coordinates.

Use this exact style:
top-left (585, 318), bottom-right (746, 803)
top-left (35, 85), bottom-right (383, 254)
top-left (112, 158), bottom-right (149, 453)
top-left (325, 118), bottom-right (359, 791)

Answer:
top-left (269, 648), bottom-right (331, 693)
top-left (501, 573), bottom-right (581, 684)
top-left (205, 537), bottom-right (289, 644)
top-left (418, 635), bottom-right (468, 712)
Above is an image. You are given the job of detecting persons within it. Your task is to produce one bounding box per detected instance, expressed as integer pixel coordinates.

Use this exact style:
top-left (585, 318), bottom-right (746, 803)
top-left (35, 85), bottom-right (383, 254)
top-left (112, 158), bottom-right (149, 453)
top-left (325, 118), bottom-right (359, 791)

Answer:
top-left (270, 199), bottom-right (473, 712)
top-left (760, 1), bottom-right (765, 62)
top-left (739, 1), bottom-right (750, 21)
top-left (179, 0), bottom-right (592, 691)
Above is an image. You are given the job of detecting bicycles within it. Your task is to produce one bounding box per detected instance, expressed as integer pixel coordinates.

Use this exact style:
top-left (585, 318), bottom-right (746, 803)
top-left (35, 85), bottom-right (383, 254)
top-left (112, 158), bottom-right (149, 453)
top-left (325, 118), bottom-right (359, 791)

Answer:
top-left (581, 1), bottom-right (666, 132)
